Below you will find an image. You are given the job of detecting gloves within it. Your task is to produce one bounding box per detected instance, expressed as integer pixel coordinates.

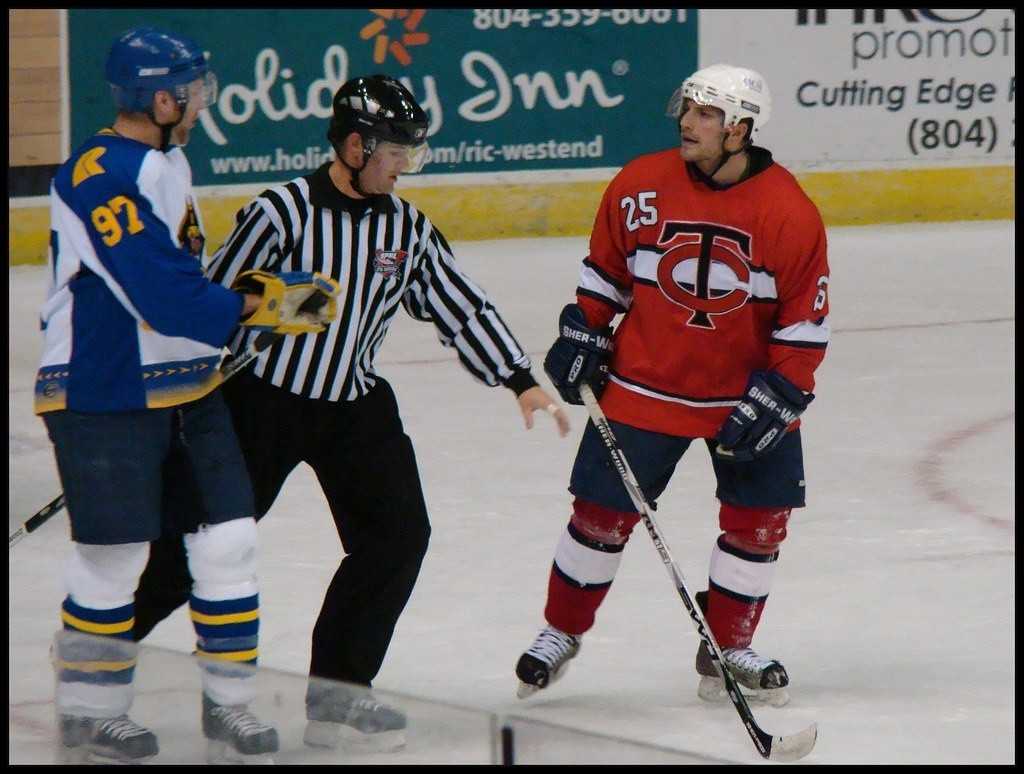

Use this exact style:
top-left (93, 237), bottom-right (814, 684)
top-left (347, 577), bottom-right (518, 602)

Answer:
top-left (544, 303), bottom-right (616, 405)
top-left (230, 268), bottom-right (341, 337)
top-left (710, 370), bottom-right (817, 464)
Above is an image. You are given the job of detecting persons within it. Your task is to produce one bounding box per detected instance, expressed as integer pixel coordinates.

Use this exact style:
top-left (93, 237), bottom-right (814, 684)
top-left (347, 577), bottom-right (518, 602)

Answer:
top-left (516, 65), bottom-right (832, 709)
top-left (133, 70), bottom-right (572, 752)
top-left (35, 31), bottom-right (342, 765)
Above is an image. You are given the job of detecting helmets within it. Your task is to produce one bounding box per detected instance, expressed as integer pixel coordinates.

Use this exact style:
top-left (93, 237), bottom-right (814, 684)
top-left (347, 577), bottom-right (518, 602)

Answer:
top-left (663, 60), bottom-right (774, 143)
top-left (102, 28), bottom-right (220, 117)
top-left (324, 75), bottom-right (430, 158)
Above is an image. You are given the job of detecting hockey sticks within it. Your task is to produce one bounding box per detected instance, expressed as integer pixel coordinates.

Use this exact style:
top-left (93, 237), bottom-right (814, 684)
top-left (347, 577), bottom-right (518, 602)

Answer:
top-left (571, 374), bottom-right (823, 764)
top-left (9, 286), bottom-right (334, 553)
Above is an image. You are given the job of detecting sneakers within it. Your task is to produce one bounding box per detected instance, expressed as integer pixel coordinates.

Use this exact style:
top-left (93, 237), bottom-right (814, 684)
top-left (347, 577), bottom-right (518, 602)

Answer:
top-left (59, 713), bottom-right (151, 768)
top-left (696, 639), bottom-right (789, 708)
top-left (301, 678), bottom-right (408, 756)
top-left (515, 620), bottom-right (584, 700)
top-left (199, 688), bottom-right (281, 767)
